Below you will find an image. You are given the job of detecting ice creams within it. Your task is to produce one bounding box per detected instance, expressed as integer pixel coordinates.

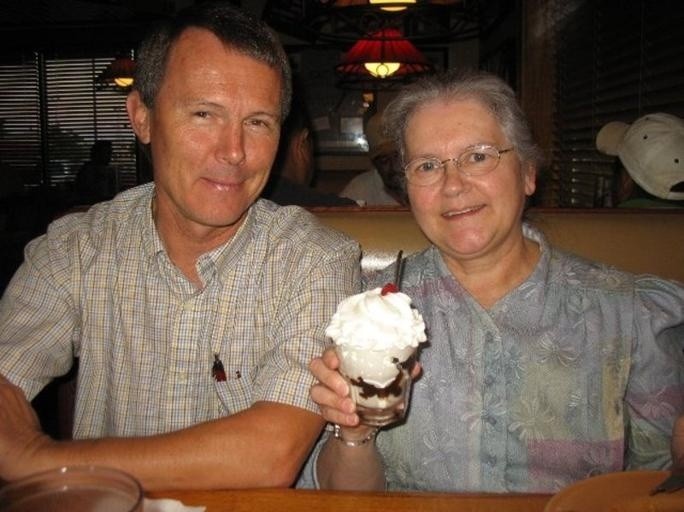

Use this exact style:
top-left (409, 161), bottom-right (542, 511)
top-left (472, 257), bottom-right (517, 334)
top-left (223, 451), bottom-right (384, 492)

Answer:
top-left (325, 283), bottom-right (428, 409)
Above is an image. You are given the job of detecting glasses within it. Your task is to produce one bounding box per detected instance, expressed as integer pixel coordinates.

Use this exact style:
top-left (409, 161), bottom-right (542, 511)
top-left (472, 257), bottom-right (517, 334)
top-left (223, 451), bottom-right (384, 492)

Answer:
top-left (402, 143), bottom-right (514, 186)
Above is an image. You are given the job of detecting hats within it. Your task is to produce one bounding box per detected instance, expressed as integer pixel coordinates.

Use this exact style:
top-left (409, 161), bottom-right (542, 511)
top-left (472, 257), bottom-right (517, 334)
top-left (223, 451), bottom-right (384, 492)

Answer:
top-left (596, 113), bottom-right (683, 201)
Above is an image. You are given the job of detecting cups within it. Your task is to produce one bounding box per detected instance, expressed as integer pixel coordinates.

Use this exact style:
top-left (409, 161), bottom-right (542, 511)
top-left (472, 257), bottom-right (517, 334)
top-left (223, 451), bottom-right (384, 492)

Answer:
top-left (335, 342), bottom-right (419, 428)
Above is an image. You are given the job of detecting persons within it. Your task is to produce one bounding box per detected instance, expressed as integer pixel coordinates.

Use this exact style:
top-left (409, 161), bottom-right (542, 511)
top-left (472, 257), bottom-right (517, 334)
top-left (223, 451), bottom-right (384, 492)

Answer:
top-left (585, 112), bottom-right (684, 211)
top-left (2, 9), bottom-right (362, 492)
top-left (292, 67), bottom-right (683, 493)
top-left (338, 97), bottom-right (405, 205)
top-left (259, 101), bottom-right (361, 209)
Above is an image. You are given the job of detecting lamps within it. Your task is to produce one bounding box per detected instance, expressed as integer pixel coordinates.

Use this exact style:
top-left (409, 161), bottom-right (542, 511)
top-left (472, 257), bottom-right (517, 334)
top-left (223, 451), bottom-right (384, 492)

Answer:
top-left (92, 56), bottom-right (140, 89)
top-left (336, 23), bottom-right (434, 82)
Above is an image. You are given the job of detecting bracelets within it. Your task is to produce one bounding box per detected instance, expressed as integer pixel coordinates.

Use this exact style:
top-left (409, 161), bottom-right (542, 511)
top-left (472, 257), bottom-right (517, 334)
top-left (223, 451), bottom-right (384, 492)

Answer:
top-left (328, 424), bottom-right (382, 448)
